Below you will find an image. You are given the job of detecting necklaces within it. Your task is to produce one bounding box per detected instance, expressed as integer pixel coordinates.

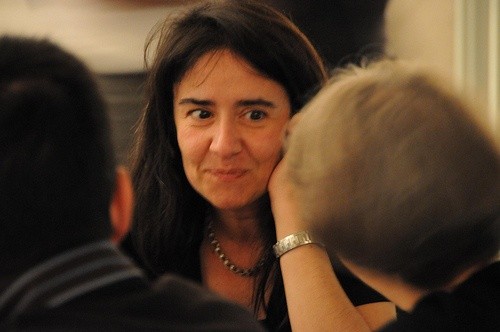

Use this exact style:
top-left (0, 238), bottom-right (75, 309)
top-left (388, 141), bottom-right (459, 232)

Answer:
top-left (208, 211), bottom-right (267, 278)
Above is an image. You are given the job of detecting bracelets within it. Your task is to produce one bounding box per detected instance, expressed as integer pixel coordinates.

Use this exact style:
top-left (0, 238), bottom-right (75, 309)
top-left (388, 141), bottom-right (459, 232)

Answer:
top-left (273, 231), bottom-right (326, 259)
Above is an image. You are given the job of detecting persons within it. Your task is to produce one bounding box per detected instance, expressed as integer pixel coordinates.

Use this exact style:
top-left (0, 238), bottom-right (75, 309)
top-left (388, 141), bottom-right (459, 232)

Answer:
top-left (282, 60), bottom-right (500, 332)
top-left (120, 2), bottom-right (394, 331)
top-left (0, 34), bottom-right (265, 332)
